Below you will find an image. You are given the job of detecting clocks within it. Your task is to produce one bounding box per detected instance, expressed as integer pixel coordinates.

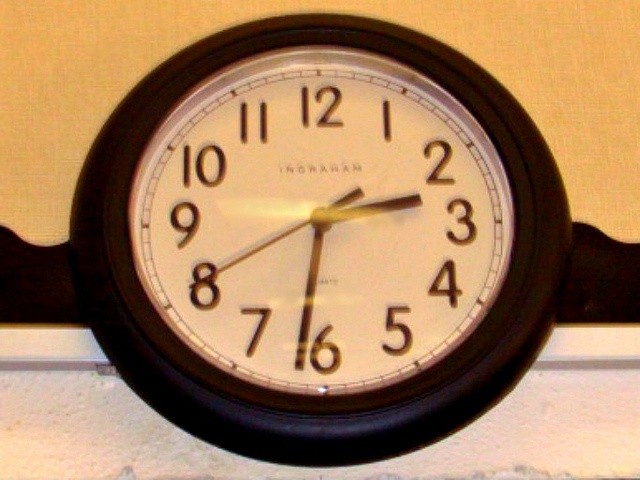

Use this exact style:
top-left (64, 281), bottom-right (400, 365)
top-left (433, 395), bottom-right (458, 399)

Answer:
top-left (66, 11), bottom-right (574, 469)
